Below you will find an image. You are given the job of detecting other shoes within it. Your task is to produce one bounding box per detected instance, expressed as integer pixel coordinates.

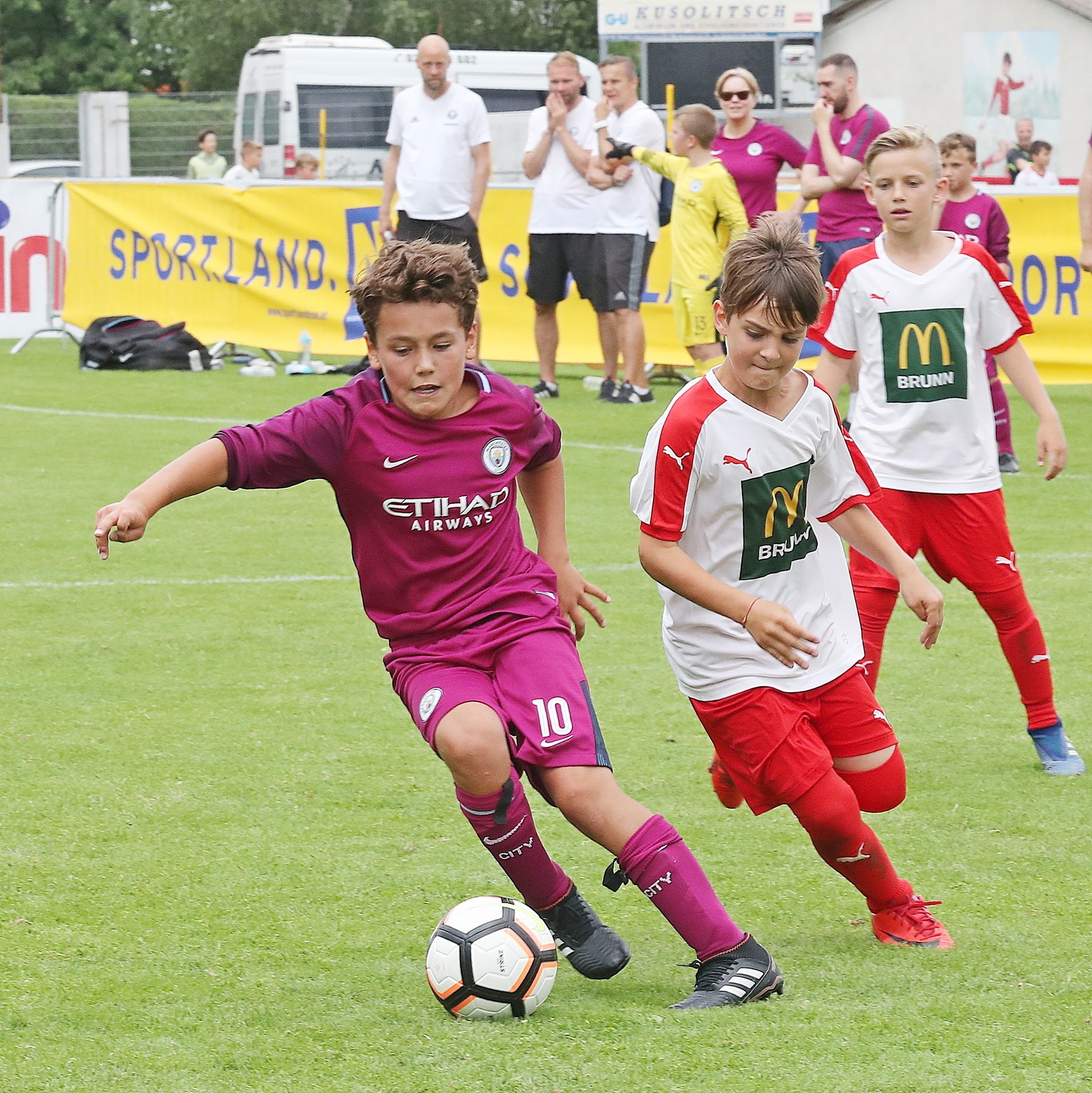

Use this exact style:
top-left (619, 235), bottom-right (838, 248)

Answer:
top-left (342, 355), bottom-right (371, 375)
top-left (842, 418), bottom-right (851, 432)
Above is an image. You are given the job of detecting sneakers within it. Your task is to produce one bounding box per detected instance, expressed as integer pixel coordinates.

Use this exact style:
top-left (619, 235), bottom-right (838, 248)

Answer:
top-left (1028, 720), bottom-right (1086, 776)
top-left (707, 749), bottom-right (744, 808)
top-left (599, 377), bottom-right (619, 402)
top-left (667, 931), bottom-right (785, 1009)
top-left (871, 895), bottom-right (956, 950)
top-left (530, 380), bottom-right (558, 400)
top-left (999, 451), bottom-right (1019, 472)
top-left (536, 883), bottom-right (630, 980)
top-left (613, 381), bottom-right (656, 404)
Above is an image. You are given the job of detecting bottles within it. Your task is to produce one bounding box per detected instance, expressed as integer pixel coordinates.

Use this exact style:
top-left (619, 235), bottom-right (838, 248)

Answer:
top-left (298, 330), bottom-right (311, 366)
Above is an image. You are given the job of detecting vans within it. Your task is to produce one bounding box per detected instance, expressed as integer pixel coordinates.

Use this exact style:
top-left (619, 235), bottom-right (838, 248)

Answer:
top-left (232, 35), bottom-right (608, 185)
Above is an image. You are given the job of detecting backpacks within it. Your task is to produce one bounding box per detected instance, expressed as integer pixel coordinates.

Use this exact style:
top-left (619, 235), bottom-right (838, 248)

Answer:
top-left (78, 316), bottom-right (212, 370)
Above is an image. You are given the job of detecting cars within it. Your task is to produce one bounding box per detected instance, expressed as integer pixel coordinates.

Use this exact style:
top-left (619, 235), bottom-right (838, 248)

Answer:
top-left (6, 161), bottom-right (82, 178)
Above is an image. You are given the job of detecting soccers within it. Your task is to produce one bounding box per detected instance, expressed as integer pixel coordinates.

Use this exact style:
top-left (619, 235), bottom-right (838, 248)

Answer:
top-left (425, 895), bottom-right (558, 1021)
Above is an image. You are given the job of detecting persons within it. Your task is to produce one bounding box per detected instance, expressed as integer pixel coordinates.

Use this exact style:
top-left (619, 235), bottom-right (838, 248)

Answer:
top-left (188, 129), bottom-right (318, 181)
top-left (979, 51), bottom-right (1030, 173)
top-left (375, 35), bottom-right (1092, 473)
top-left (798, 129), bottom-right (1086, 775)
top-left (629, 218), bottom-right (952, 950)
top-left (92, 238), bottom-right (783, 1010)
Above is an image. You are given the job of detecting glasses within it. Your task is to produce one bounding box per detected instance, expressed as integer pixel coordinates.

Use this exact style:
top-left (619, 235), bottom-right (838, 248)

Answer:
top-left (717, 89), bottom-right (755, 100)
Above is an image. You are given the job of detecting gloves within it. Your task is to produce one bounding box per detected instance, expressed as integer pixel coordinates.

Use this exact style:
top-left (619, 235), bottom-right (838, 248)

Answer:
top-left (604, 138), bottom-right (635, 159)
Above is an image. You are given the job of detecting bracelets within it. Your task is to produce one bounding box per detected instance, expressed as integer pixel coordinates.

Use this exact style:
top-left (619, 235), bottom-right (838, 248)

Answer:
top-left (595, 120), bottom-right (609, 132)
top-left (742, 597), bottom-right (761, 628)
